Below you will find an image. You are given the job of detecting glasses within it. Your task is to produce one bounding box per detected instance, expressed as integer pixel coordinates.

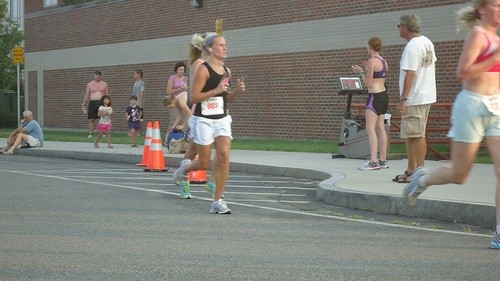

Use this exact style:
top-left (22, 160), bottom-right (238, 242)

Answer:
top-left (397, 24), bottom-right (400, 28)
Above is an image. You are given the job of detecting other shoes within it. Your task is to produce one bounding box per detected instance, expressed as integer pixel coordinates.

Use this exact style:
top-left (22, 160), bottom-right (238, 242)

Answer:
top-left (94, 142), bottom-right (99, 148)
top-left (108, 144), bottom-right (113, 147)
top-left (3, 149), bottom-right (15, 155)
top-left (0, 148), bottom-right (7, 152)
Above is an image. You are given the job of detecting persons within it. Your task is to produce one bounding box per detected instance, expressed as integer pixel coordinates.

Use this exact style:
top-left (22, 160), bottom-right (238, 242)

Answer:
top-left (351, 36), bottom-right (390, 171)
top-left (128, 69), bottom-right (146, 137)
top-left (392, 12), bottom-right (437, 184)
top-left (399, 0), bottom-right (499, 249)
top-left (125, 94), bottom-right (143, 147)
top-left (93, 95), bottom-right (114, 148)
top-left (172, 32), bottom-right (245, 215)
top-left (81, 70), bottom-right (109, 138)
top-left (165, 31), bottom-right (226, 201)
top-left (0, 110), bottom-right (44, 154)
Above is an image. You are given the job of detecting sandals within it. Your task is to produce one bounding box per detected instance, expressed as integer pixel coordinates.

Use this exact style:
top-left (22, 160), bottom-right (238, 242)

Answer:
top-left (392, 170), bottom-right (412, 182)
top-left (398, 177), bottom-right (412, 183)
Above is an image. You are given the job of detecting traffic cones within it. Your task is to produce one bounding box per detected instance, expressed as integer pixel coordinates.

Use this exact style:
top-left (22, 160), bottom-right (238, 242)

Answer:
top-left (135, 121), bottom-right (153, 166)
top-left (142, 120), bottom-right (169, 172)
top-left (187, 149), bottom-right (209, 185)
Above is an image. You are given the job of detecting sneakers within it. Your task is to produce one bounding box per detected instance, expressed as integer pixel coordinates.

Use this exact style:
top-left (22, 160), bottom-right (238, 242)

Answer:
top-left (489, 232), bottom-right (500, 249)
top-left (176, 176), bottom-right (191, 199)
top-left (378, 160), bottom-right (389, 169)
top-left (358, 160), bottom-right (380, 170)
top-left (210, 199), bottom-right (231, 214)
top-left (173, 159), bottom-right (192, 183)
top-left (205, 181), bottom-right (224, 198)
top-left (402, 166), bottom-right (426, 207)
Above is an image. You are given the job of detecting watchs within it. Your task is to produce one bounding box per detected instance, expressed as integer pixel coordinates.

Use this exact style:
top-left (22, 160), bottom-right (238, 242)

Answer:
top-left (400, 95), bottom-right (408, 101)
